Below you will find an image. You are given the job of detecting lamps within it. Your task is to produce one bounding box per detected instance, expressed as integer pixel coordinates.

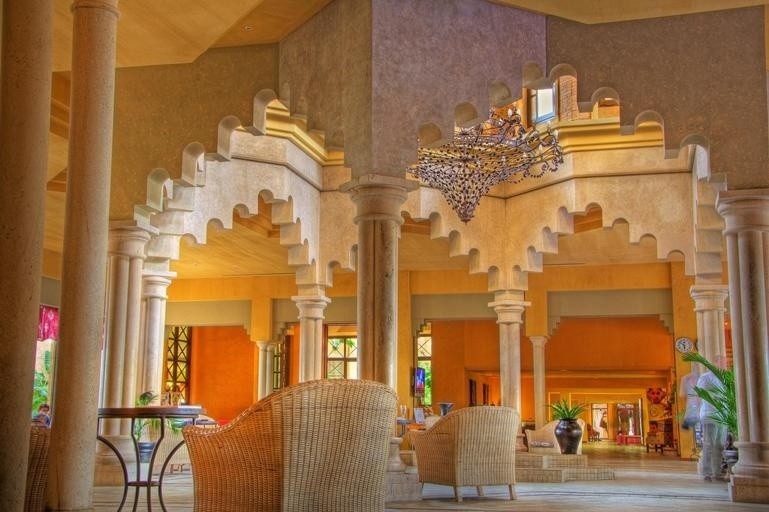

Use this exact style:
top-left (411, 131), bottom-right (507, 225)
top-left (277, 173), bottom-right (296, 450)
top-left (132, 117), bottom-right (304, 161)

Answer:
top-left (406, 101), bottom-right (566, 228)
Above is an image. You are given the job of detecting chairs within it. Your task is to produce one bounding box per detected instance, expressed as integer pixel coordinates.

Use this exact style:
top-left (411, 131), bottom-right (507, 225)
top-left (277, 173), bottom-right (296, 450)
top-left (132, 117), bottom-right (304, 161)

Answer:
top-left (23, 419), bottom-right (51, 512)
top-left (412, 403), bottom-right (522, 504)
top-left (149, 415), bottom-right (221, 473)
top-left (524, 418), bottom-right (585, 455)
top-left (183, 377), bottom-right (400, 511)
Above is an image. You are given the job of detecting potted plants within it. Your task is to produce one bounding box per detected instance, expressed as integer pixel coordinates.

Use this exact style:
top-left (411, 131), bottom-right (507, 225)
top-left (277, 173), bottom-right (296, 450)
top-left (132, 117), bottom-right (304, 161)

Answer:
top-left (132, 390), bottom-right (183, 463)
top-left (542, 396), bottom-right (590, 455)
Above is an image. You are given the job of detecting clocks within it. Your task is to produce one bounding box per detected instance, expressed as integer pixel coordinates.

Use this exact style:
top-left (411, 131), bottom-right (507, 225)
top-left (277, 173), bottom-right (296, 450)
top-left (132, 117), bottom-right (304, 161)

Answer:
top-left (675, 336), bottom-right (694, 354)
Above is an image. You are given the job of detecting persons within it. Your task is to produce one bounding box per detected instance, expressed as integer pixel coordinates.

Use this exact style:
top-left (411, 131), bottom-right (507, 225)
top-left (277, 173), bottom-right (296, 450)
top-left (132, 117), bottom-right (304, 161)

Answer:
top-left (587, 424), bottom-right (602, 442)
top-left (32, 403), bottom-right (51, 432)
top-left (696, 354), bottom-right (729, 484)
top-left (650, 422), bottom-right (664, 443)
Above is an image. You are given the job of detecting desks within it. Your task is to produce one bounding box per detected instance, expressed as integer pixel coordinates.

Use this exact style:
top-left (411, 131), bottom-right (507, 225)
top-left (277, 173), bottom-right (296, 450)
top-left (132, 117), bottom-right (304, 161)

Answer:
top-left (97, 407), bottom-right (202, 512)
